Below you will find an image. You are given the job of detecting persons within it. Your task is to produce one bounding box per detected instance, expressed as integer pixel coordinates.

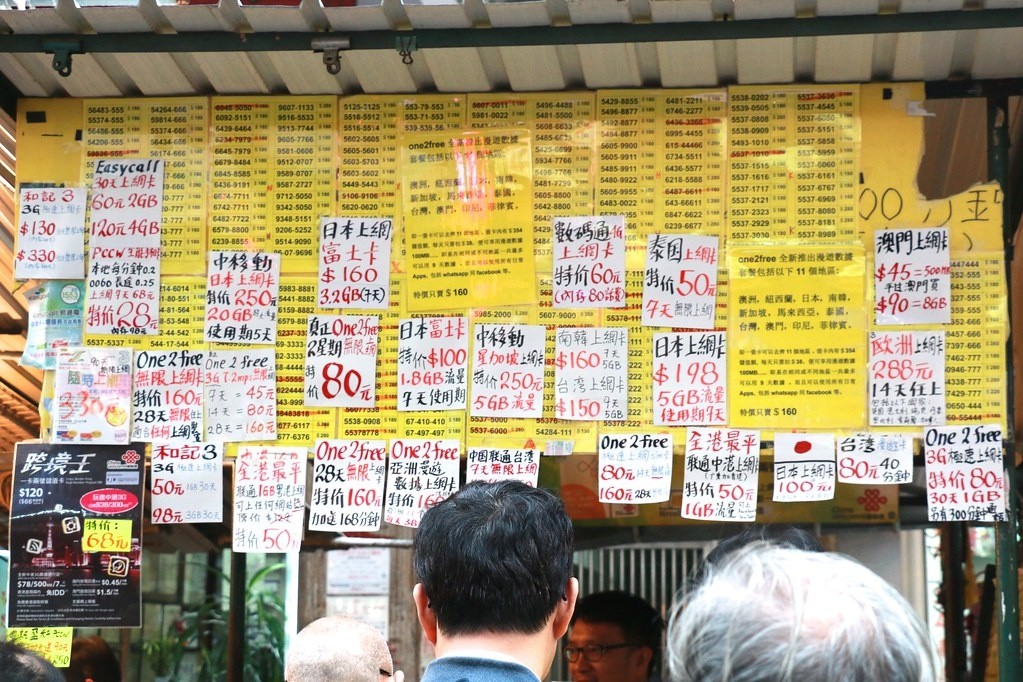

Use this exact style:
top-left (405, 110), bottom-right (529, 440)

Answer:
top-left (285, 614), bottom-right (404, 682)
top-left (0, 635), bottom-right (122, 682)
top-left (666, 525), bottom-right (943, 682)
top-left (412, 481), bottom-right (579, 682)
top-left (967, 574), bottom-right (998, 681)
top-left (566, 591), bottom-right (667, 682)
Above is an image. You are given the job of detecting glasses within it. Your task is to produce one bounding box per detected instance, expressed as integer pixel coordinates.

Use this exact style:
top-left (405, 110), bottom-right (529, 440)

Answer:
top-left (564, 643), bottom-right (639, 662)
